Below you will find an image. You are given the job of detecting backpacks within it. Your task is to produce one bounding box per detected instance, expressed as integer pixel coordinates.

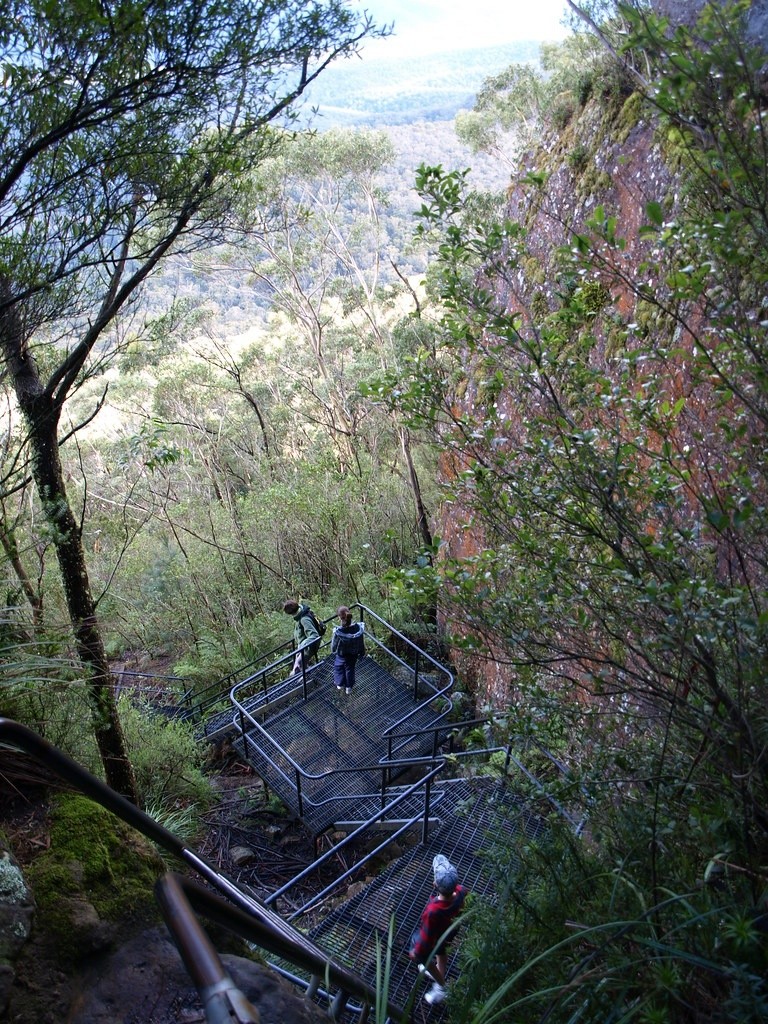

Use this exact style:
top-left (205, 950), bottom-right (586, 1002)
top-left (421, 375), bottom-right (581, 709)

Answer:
top-left (299, 610), bottom-right (327, 636)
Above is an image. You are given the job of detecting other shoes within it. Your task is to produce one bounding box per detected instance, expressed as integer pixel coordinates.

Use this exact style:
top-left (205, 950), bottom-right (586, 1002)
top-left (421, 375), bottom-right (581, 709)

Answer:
top-left (417, 963), bottom-right (436, 982)
top-left (345, 687), bottom-right (352, 694)
top-left (336, 685), bottom-right (343, 689)
top-left (424, 982), bottom-right (455, 1005)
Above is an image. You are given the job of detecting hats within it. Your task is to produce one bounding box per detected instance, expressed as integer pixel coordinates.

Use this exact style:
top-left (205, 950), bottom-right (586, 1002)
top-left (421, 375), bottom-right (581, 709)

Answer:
top-left (432, 853), bottom-right (459, 892)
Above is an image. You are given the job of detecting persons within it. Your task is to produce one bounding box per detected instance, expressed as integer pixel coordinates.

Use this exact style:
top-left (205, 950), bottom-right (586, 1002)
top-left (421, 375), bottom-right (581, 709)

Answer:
top-left (283, 601), bottom-right (327, 687)
top-left (409, 854), bottom-right (468, 1005)
top-left (331, 605), bottom-right (365, 694)
